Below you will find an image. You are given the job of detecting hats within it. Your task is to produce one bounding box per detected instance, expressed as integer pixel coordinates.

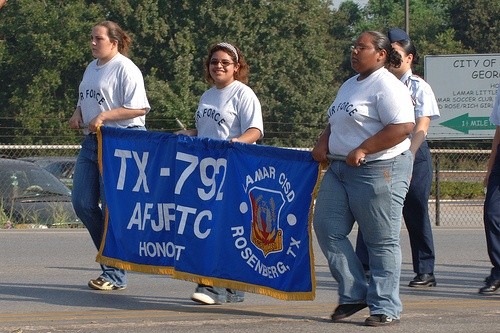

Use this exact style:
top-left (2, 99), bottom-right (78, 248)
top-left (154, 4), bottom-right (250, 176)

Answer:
top-left (387, 28), bottom-right (407, 42)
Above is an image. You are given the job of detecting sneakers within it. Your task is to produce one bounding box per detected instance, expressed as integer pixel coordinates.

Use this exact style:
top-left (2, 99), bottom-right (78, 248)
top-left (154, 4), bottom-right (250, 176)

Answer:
top-left (88, 275), bottom-right (127, 291)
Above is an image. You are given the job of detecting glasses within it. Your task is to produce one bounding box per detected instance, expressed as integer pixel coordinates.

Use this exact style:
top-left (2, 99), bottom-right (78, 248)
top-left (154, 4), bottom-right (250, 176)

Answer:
top-left (210, 60), bottom-right (235, 66)
top-left (351, 45), bottom-right (374, 51)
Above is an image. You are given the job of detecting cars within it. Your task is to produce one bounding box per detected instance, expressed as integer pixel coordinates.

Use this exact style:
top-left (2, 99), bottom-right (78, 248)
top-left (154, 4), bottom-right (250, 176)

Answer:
top-left (0, 158), bottom-right (102, 229)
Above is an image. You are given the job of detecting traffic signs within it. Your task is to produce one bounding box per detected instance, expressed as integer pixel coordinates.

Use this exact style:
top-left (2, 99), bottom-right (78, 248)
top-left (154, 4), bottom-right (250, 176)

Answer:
top-left (424, 54), bottom-right (500, 141)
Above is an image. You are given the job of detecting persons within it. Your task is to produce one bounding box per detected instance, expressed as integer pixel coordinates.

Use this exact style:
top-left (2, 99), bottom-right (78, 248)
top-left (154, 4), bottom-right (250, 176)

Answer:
top-left (69, 20), bottom-right (151, 290)
top-left (312, 31), bottom-right (416, 327)
top-left (174, 42), bottom-right (264, 305)
top-left (479, 85), bottom-right (500, 294)
top-left (355, 29), bottom-right (441, 288)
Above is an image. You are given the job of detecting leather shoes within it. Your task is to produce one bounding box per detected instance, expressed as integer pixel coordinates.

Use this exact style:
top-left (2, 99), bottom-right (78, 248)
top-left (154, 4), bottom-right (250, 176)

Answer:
top-left (365, 273), bottom-right (371, 280)
top-left (409, 273), bottom-right (436, 287)
top-left (480, 279), bottom-right (500, 294)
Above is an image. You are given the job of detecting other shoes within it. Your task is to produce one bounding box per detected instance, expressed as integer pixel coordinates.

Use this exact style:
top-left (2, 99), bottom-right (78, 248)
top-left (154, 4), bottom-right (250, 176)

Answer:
top-left (190, 292), bottom-right (225, 304)
top-left (227, 295), bottom-right (244, 301)
top-left (331, 303), bottom-right (367, 319)
top-left (365, 314), bottom-right (400, 325)
